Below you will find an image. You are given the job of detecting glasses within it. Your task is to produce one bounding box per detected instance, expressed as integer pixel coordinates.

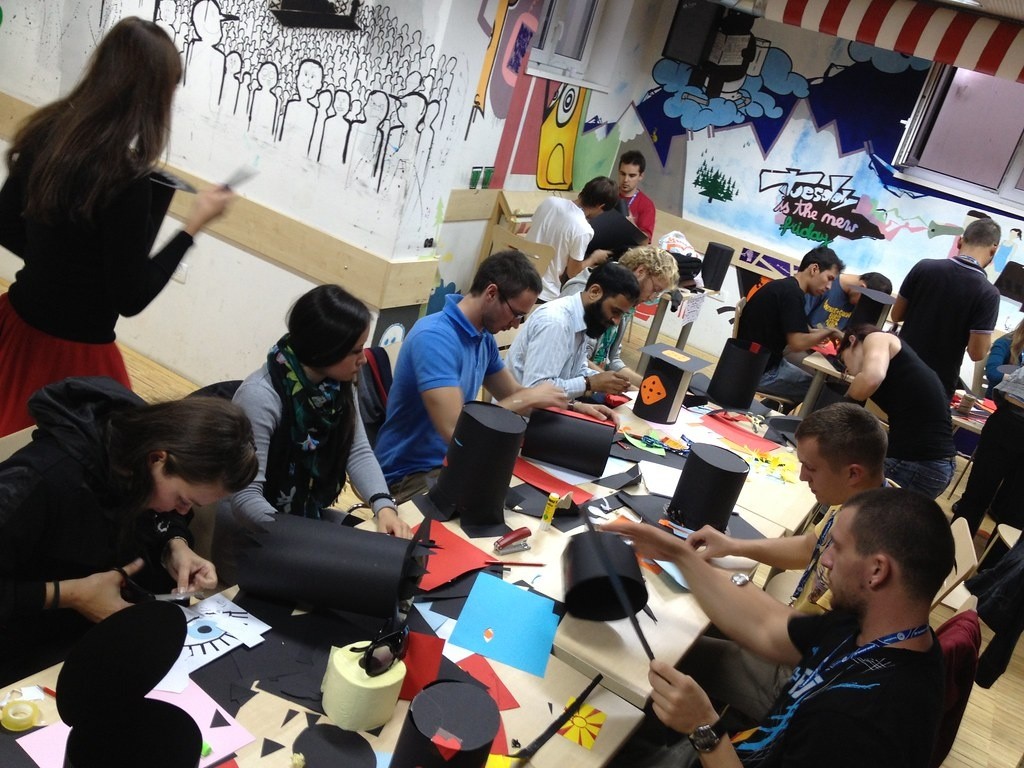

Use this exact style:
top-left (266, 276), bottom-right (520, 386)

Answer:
top-left (651, 277), bottom-right (661, 297)
top-left (350, 618), bottom-right (410, 677)
top-left (502, 292), bottom-right (523, 320)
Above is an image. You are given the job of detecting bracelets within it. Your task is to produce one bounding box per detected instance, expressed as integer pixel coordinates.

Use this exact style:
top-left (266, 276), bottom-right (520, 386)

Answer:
top-left (49, 580), bottom-right (60, 613)
top-left (584, 376), bottom-right (591, 391)
top-left (368, 493), bottom-right (396, 507)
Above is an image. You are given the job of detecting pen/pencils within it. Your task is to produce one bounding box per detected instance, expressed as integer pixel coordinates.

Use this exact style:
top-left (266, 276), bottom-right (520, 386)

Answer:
top-left (36, 684), bottom-right (57, 697)
top-left (617, 441), bottom-right (627, 450)
top-left (484, 560), bottom-right (546, 567)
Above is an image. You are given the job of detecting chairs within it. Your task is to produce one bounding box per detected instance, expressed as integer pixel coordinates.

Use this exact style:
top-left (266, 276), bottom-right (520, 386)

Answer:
top-left (2, 224), bottom-right (568, 605)
top-left (925, 515), bottom-right (1024, 768)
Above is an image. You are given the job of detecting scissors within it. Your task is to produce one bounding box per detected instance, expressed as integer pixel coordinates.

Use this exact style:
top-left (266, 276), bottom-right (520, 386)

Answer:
top-left (114, 567), bottom-right (206, 604)
top-left (746, 412), bottom-right (765, 433)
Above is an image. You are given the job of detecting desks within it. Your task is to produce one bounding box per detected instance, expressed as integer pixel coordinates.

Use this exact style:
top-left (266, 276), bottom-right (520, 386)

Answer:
top-left (633, 285), bottom-right (726, 378)
top-left (800, 348), bottom-right (995, 442)
top-left (0, 89), bottom-right (437, 312)
top-left (0, 379), bottom-right (824, 768)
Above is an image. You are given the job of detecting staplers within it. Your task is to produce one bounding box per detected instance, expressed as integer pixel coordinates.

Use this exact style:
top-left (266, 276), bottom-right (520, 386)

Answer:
top-left (493, 526), bottom-right (532, 554)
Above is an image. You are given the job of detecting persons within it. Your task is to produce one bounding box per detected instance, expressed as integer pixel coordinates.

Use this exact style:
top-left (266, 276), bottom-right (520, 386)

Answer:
top-left (0, 375), bottom-right (260, 688)
top-left (0, 16), bottom-right (233, 440)
top-left (602, 486), bottom-right (956, 768)
top-left (736, 218), bottom-right (1024, 574)
top-left (685, 402), bottom-right (902, 615)
top-left (373, 252), bottom-right (621, 505)
top-left (524, 150), bottom-right (679, 390)
top-left (490, 261), bottom-right (640, 404)
top-left (211, 284), bottom-right (414, 587)
top-left (839, 323), bottom-right (956, 500)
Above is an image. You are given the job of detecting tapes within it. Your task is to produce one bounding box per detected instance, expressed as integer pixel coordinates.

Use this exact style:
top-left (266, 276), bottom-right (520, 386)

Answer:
top-left (1, 700), bottom-right (39, 732)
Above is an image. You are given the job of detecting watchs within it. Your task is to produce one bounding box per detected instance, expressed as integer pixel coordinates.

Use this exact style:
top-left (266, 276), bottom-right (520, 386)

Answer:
top-left (730, 572), bottom-right (749, 587)
top-left (688, 719), bottom-right (730, 753)
